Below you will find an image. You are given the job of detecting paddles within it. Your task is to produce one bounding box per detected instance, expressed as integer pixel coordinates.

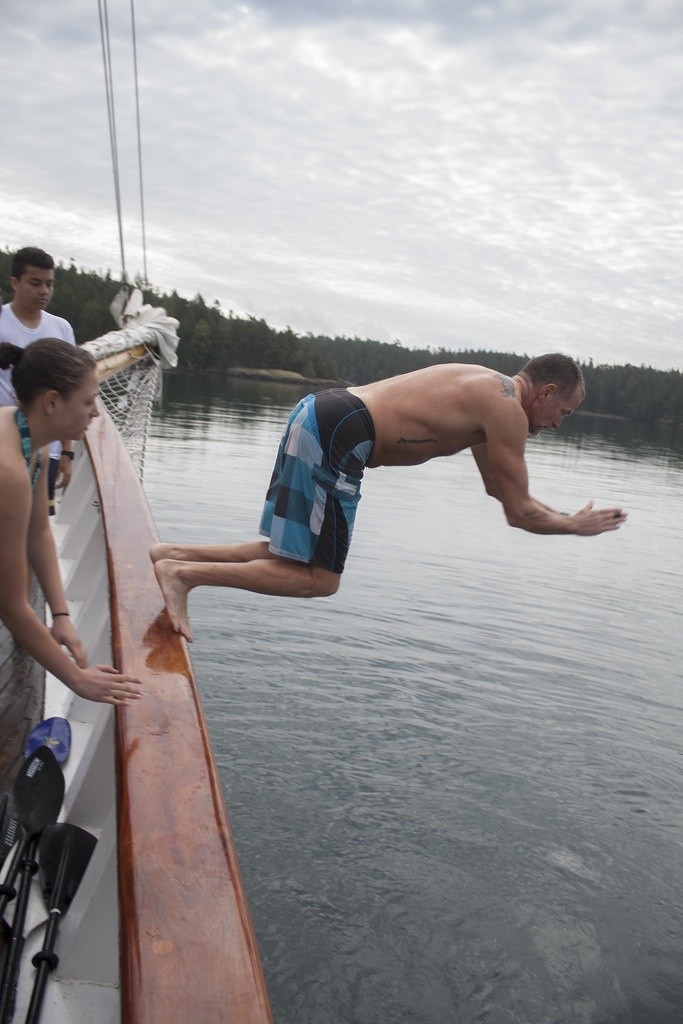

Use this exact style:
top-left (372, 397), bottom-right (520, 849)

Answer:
top-left (0, 715), bottom-right (73, 1024)
top-left (22, 821), bottom-right (99, 1024)
top-left (0, 746), bottom-right (66, 917)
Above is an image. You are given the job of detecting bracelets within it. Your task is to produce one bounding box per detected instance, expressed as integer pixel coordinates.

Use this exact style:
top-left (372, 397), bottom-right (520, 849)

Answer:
top-left (53, 613), bottom-right (68, 617)
top-left (61, 451), bottom-right (75, 460)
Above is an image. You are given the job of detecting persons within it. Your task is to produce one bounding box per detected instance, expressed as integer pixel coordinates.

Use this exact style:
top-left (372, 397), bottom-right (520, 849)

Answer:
top-left (0, 337), bottom-right (144, 707)
top-left (0, 247), bottom-right (74, 515)
top-left (148, 353), bottom-right (628, 644)
